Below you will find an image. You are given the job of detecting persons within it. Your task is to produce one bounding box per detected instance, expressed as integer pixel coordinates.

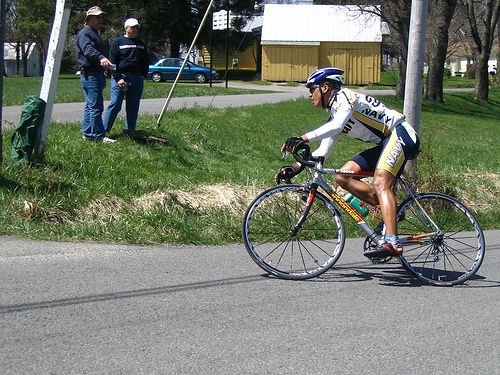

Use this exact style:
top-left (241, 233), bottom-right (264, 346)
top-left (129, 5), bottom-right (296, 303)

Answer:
top-left (103, 18), bottom-right (150, 137)
top-left (76, 6), bottom-right (117, 143)
top-left (490, 65), bottom-right (497, 77)
top-left (275, 67), bottom-right (419, 257)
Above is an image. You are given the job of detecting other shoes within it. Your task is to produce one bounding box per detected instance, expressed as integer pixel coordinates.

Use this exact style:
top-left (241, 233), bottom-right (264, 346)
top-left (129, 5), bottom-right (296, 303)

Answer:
top-left (82, 136), bottom-right (92, 140)
top-left (96, 136), bottom-right (118, 144)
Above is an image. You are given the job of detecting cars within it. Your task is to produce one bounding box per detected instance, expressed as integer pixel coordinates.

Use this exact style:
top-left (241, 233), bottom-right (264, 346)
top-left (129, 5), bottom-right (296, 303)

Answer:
top-left (146, 57), bottom-right (220, 84)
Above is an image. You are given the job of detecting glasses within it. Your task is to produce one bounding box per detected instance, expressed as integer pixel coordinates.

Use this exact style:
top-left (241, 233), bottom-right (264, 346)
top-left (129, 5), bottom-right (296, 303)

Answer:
top-left (310, 84), bottom-right (325, 93)
top-left (92, 14), bottom-right (105, 19)
top-left (128, 25), bottom-right (138, 29)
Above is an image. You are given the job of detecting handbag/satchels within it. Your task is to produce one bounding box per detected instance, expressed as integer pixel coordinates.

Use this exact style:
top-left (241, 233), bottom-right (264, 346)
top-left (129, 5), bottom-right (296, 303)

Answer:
top-left (11, 95), bottom-right (47, 166)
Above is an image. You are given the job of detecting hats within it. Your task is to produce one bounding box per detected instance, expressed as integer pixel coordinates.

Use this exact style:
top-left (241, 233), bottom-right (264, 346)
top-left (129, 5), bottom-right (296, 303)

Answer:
top-left (124, 18), bottom-right (141, 28)
top-left (86, 6), bottom-right (106, 16)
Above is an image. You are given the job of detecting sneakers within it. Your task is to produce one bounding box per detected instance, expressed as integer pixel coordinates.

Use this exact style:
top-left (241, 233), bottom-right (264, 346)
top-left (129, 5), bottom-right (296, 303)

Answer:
top-left (363, 239), bottom-right (403, 258)
top-left (374, 208), bottom-right (405, 235)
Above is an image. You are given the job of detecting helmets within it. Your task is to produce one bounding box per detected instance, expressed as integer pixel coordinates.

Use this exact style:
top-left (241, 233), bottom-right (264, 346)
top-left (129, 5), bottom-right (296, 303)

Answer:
top-left (306, 67), bottom-right (345, 89)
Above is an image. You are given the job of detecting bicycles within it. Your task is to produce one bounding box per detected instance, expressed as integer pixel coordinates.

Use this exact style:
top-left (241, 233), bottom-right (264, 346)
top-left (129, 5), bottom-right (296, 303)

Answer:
top-left (242, 142), bottom-right (485, 288)
